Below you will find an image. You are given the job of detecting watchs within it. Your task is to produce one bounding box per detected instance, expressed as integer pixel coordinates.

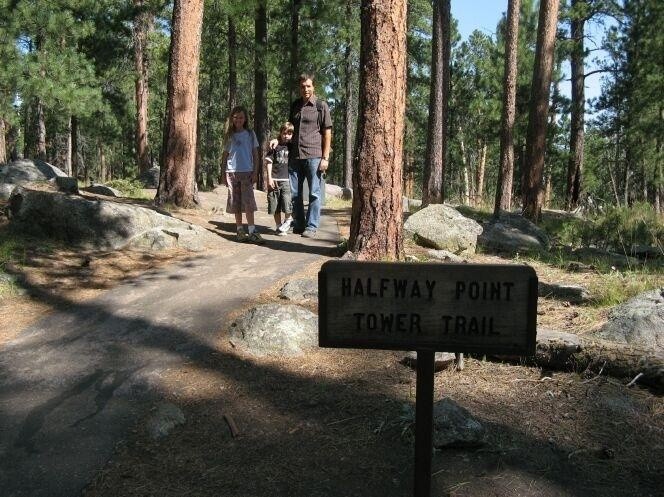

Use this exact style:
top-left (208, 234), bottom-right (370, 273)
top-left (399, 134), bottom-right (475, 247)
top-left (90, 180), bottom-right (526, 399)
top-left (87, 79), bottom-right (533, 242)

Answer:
top-left (322, 157), bottom-right (329, 161)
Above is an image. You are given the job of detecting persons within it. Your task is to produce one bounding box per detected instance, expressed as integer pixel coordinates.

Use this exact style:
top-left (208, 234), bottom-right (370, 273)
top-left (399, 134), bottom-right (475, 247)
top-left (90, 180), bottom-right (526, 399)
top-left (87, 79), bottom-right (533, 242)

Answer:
top-left (266, 71), bottom-right (333, 238)
top-left (264, 121), bottom-right (294, 236)
top-left (217, 106), bottom-right (266, 244)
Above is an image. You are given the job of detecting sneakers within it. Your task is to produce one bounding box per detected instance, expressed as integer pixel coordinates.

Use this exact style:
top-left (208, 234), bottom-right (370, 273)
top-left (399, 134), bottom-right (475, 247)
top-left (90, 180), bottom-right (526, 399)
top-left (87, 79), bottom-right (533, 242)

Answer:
top-left (237, 221), bottom-right (316, 245)
